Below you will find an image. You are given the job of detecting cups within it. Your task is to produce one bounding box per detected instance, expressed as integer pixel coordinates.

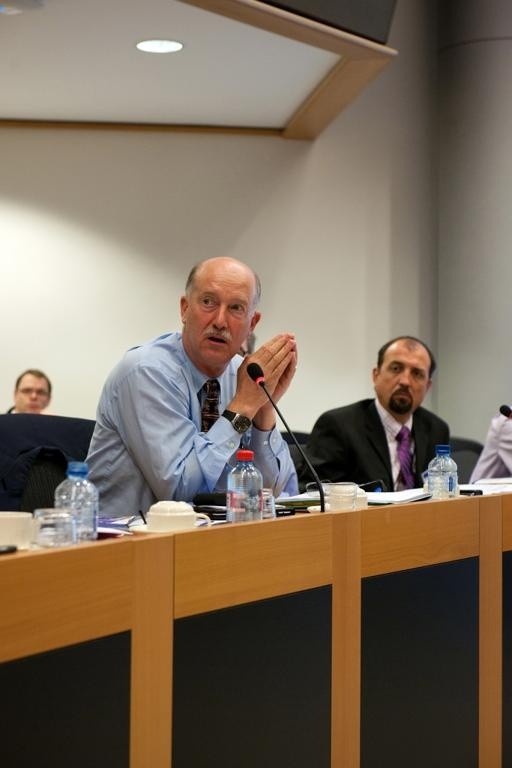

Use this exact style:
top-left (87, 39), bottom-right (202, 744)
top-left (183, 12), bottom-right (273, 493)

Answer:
top-left (147, 500), bottom-right (211, 532)
top-left (30, 508), bottom-right (78, 553)
top-left (261, 487), bottom-right (277, 521)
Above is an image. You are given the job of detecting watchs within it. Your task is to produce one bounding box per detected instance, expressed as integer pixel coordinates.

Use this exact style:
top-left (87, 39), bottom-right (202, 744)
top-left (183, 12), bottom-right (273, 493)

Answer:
top-left (221, 409), bottom-right (251, 434)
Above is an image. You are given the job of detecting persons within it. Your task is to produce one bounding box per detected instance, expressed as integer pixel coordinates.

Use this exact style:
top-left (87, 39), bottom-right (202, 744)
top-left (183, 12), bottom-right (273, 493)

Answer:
top-left (468, 405), bottom-right (512, 484)
top-left (297, 335), bottom-right (450, 497)
top-left (6, 369), bottom-right (52, 414)
top-left (77, 256), bottom-right (299, 516)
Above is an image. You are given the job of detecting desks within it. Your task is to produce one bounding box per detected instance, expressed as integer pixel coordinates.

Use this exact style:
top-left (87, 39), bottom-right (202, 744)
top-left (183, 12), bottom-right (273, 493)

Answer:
top-left (1, 493), bottom-right (512, 768)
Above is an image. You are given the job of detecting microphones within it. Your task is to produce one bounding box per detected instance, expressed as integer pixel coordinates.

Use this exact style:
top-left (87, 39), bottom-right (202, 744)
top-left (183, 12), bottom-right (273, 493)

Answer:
top-left (499, 404), bottom-right (512, 419)
top-left (246, 363), bottom-right (325, 513)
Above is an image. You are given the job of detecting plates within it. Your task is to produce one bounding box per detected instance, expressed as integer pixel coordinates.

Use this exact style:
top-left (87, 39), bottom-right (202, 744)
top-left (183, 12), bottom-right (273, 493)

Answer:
top-left (306, 504), bottom-right (322, 513)
top-left (129, 524), bottom-right (171, 535)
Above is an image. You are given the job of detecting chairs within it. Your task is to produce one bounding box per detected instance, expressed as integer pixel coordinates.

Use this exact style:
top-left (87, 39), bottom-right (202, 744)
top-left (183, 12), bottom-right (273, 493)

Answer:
top-left (1, 414), bottom-right (97, 510)
top-left (279, 430), bottom-right (311, 479)
top-left (448, 437), bottom-right (483, 484)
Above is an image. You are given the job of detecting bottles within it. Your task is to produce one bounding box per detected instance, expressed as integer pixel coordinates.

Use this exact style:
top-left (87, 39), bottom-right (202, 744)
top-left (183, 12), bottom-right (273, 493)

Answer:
top-left (326, 482), bottom-right (357, 511)
top-left (225, 449), bottom-right (263, 525)
top-left (53, 461), bottom-right (102, 544)
top-left (426, 445), bottom-right (460, 502)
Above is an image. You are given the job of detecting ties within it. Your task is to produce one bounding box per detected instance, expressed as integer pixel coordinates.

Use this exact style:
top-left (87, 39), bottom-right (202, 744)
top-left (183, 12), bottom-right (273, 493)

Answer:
top-left (396, 424), bottom-right (426, 484)
top-left (199, 375), bottom-right (223, 433)
